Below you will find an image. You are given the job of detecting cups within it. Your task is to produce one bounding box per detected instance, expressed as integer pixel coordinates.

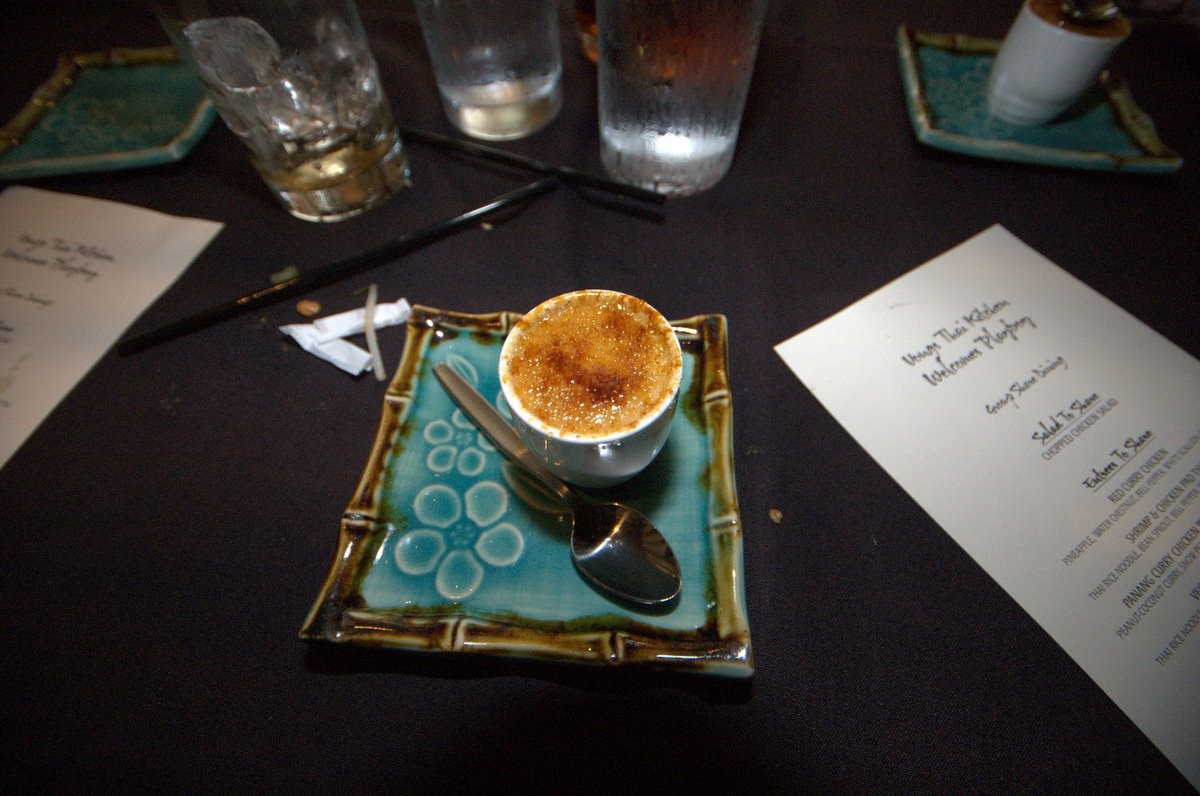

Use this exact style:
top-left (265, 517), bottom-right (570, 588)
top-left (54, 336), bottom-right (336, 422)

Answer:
top-left (589, 0), bottom-right (775, 196)
top-left (416, 0), bottom-right (571, 141)
top-left (142, 1), bottom-right (413, 222)
top-left (497, 289), bottom-right (689, 492)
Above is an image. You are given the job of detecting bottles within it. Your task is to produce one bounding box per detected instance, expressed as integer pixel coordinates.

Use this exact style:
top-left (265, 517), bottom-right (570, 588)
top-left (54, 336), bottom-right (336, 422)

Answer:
top-left (981, 0), bottom-right (1135, 125)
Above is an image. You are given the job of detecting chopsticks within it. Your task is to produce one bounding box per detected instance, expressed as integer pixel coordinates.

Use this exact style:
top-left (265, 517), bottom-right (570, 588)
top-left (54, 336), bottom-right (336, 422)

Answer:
top-left (115, 112), bottom-right (684, 358)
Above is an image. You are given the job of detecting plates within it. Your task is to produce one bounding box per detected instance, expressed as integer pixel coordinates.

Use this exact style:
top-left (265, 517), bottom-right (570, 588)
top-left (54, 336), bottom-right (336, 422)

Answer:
top-left (0, 43), bottom-right (225, 187)
top-left (292, 299), bottom-right (755, 680)
top-left (892, 20), bottom-right (1186, 175)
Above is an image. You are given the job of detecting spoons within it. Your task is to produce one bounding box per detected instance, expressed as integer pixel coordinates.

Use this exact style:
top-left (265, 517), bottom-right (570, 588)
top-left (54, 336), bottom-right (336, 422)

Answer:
top-left (432, 364), bottom-right (685, 608)
